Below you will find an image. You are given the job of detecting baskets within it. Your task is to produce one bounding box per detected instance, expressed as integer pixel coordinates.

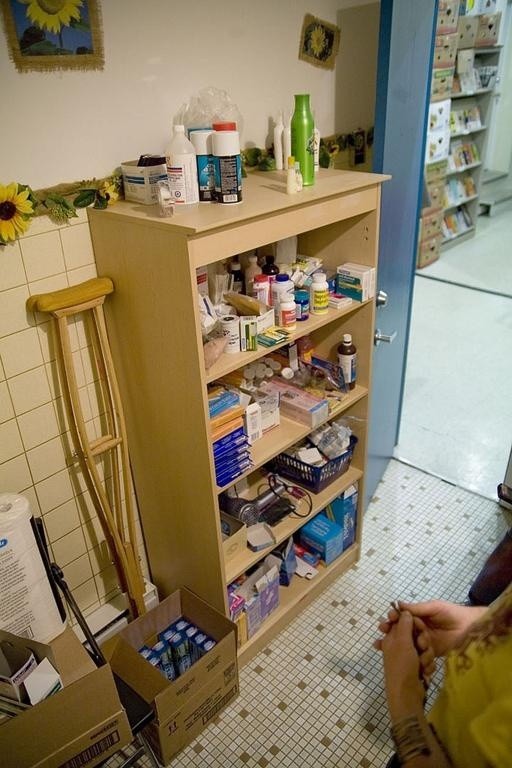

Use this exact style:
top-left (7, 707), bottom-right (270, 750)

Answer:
top-left (262, 420), bottom-right (358, 495)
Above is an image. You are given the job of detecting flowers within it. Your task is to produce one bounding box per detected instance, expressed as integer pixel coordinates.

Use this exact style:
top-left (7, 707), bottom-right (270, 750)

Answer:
top-left (0, 181), bottom-right (75, 246)
top-left (328, 144), bottom-right (339, 157)
top-left (75, 177), bottom-right (118, 209)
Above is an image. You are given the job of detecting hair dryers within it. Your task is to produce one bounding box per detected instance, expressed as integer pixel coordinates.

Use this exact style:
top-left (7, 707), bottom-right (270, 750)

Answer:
top-left (220, 484), bottom-right (286, 527)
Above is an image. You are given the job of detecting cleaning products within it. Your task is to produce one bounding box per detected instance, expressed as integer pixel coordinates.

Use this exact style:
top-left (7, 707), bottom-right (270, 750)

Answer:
top-left (273, 108), bottom-right (283, 172)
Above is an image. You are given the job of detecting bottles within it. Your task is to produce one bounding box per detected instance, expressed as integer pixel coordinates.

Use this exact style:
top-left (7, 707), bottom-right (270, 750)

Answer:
top-left (278, 293), bottom-right (297, 329)
top-left (290, 92), bottom-right (315, 185)
top-left (336, 334), bottom-right (357, 390)
top-left (298, 335), bottom-right (313, 365)
top-left (311, 273), bottom-right (329, 314)
top-left (285, 157), bottom-right (304, 194)
top-left (274, 109), bottom-right (320, 172)
top-left (229, 256), bottom-right (296, 325)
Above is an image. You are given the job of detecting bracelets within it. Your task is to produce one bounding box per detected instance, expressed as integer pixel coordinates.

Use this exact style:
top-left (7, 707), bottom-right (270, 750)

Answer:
top-left (389, 714), bottom-right (453, 764)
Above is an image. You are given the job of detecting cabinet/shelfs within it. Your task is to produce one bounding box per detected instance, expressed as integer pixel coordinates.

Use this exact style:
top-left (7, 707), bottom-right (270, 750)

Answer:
top-left (441, 46), bottom-right (503, 254)
top-left (84, 170), bottom-right (390, 673)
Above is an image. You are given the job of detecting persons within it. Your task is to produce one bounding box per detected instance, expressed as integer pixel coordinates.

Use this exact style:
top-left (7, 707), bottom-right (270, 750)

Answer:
top-left (373, 581), bottom-right (512, 768)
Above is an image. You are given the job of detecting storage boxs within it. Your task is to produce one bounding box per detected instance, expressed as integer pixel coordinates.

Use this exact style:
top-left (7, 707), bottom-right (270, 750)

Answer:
top-left (101, 588), bottom-right (238, 766)
top-left (458, 15), bottom-right (476, 48)
top-left (417, 1), bottom-right (462, 269)
top-left (0, 627), bottom-right (133, 768)
top-left (476, 14), bottom-right (501, 47)
